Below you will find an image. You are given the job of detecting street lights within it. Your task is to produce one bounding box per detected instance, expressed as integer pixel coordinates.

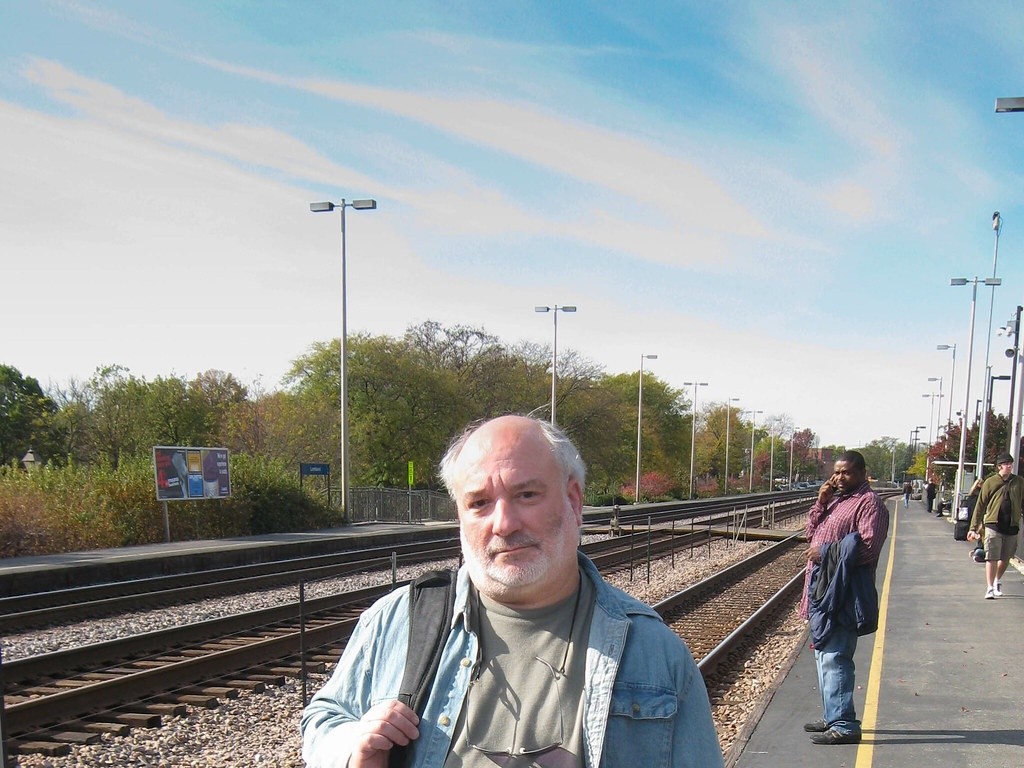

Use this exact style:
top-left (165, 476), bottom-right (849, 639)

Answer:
top-left (636, 353), bottom-right (657, 503)
top-left (725, 397), bottom-right (740, 496)
top-left (920, 393), bottom-right (944, 481)
top-left (767, 418), bottom-right (782, 491)
top-left (784, 427), bottom-right (799, 489)
top-left (928, 376), bottom-right (943, 445)
top-left (745, 410), bottom-right (763, 493)
top-left (310, 198), bottom-right (378, 522)
top-left (534, 304), bottom-right (576, 425)
top-left (951, 276), bottom-right (1001, 516)
top-left (683, 381), bottom-right (708, 496)
top-left (936, 343), bottom-right (956, 452)
top-left (908, 426), bottom-right (927, 456)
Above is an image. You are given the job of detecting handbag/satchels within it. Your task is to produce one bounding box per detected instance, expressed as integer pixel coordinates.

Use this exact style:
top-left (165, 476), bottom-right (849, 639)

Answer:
top-left (969, 539), bottom-right (986, 562)
top-left (959, 507), bottom-right (968, 520)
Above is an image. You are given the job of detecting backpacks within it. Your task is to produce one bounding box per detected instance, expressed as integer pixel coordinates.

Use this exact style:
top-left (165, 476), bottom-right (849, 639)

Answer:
top-left (956, 494), bottom-right (978, 522)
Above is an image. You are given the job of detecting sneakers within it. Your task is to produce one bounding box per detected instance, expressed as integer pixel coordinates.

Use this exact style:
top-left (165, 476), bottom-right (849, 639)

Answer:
top-left (803, 720), bottom-right (830, 732)
top-left (810, 727), bottom-right (861, 745)
top-left (993, 581), bottom-right (1003, 596)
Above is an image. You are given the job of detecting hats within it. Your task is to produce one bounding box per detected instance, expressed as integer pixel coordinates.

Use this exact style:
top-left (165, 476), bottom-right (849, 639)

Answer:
top-left (996, 453), bottom-right (1014, 463)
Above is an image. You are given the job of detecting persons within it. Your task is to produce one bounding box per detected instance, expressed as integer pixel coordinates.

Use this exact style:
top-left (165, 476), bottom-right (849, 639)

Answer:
top-left (926, 478), bottom-right (935, 513)
top-left (936, 490), bottom-right (954, 517)
top-left (298, 406), bottom-right (727, 768)
top-left (968, 476), bottom-right (985, 530)
top-left (967, 452), bottom-right (1024, 599)
top-left (902, 483), bottom-right (912, 508)
top-left (799, 448), bottom-right (890, 746)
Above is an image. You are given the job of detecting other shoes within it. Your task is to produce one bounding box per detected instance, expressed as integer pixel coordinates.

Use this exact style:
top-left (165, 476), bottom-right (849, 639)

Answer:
top-left (937, 514), bottom-right (944, 517)
top-left (984, 587), bottom-right (995, 599)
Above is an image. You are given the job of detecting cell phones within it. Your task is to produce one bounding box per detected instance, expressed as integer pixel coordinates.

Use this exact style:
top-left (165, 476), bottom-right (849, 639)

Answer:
top-left (831, 480), bottom-right (839, 493)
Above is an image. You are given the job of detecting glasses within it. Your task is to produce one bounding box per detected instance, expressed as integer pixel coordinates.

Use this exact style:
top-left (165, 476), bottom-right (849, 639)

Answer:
top-left (465, 656), bottom-right (564, 757)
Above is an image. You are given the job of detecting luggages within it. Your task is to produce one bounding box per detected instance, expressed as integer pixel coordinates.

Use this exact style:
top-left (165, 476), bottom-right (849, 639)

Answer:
top-left (955, 508), bottom-right (969, 541)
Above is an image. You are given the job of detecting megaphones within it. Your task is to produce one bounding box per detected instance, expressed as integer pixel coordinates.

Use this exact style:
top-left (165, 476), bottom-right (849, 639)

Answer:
top-left (1005, 349), bottom-right (1016, 358)
